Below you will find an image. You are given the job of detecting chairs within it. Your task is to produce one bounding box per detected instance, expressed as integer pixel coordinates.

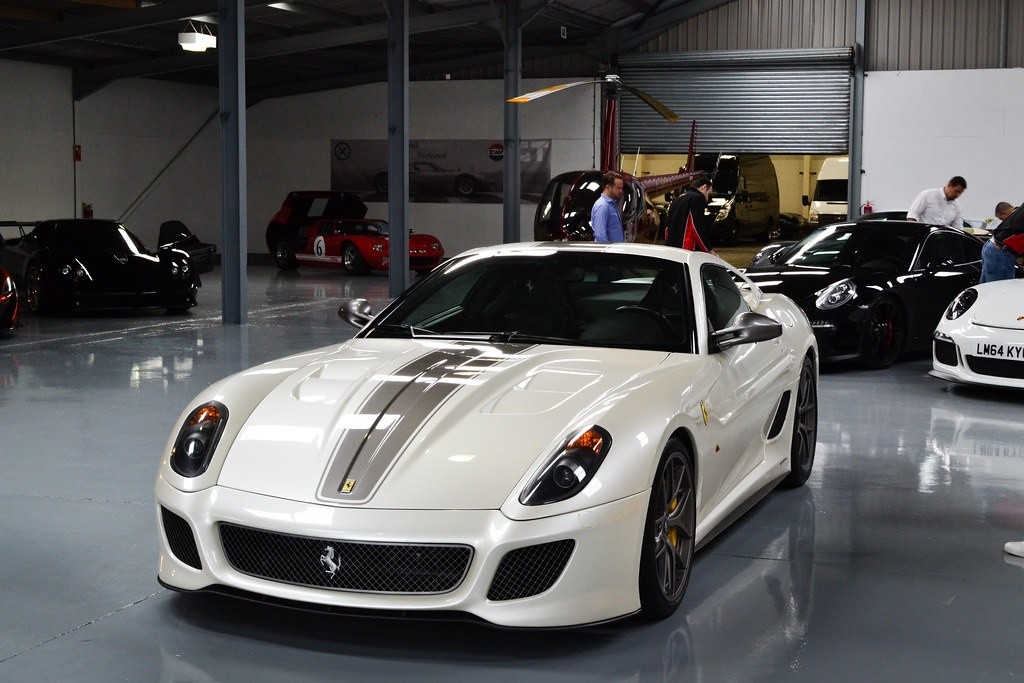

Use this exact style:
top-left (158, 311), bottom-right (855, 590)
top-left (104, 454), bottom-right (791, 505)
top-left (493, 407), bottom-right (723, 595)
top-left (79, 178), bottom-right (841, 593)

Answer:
top-left (476, 276), bottom-right (713, 343)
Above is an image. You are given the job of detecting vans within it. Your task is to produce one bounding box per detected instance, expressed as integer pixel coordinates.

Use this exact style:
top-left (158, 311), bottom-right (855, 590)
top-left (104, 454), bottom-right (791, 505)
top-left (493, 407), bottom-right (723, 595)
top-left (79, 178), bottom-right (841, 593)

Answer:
top-left (663, 152), bottom-right (781, 243)
top-left (801, 157), bottom-right (851, 229)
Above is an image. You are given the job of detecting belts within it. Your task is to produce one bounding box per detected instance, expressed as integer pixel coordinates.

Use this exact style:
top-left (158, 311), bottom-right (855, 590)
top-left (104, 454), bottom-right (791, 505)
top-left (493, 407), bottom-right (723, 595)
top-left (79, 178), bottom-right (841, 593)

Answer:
top-left (990, 237), bottom-right (1020, 258)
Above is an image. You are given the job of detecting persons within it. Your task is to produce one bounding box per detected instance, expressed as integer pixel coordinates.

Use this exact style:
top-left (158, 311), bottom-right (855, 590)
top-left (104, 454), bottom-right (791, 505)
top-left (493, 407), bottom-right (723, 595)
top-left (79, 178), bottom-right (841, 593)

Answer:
top-left (995, 200), bottom-right (1014, 221)
top-left (978, 200), bottom-right (1024, 281)
top-left (662, 176), bottom-right (719, 257)
top-left (905, 176), bottom-right (968, 230)
top-left (592, 170), bottom-right (627, 242)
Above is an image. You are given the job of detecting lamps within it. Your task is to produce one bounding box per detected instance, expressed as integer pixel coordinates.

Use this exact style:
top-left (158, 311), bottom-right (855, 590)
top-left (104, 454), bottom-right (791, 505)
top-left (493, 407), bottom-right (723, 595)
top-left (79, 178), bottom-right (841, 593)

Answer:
top-left (179, 20), bottom-right (217, 52)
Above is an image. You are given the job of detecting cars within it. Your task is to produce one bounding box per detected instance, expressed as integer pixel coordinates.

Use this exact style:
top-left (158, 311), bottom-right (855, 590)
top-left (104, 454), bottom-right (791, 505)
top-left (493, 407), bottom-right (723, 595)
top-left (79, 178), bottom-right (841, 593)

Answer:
top-left (407, 161), bottom-right (484, 197)
top-left (149, 239), bottom-right (824, 633)
top-left (302, 215), bottom-right (446, 276)
top-left (739, 219), bottom-right (991, 363)
top-left (0, 235), bottom-right (20, 337)
top-left (19, 218), bottom-right (198, 318)
top-left (927, 275), bottom-right (1024, 394)
